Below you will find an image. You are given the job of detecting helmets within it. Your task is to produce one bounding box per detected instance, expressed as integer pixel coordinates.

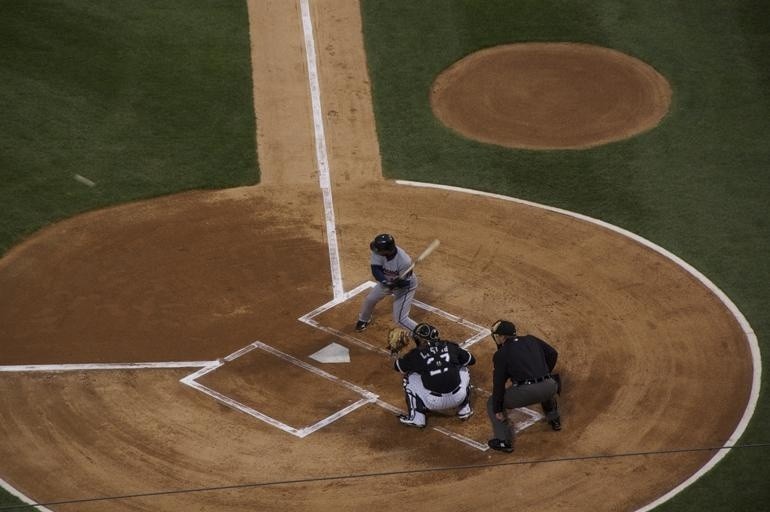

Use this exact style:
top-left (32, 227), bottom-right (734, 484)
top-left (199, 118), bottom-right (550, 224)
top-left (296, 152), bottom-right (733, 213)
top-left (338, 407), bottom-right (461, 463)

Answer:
top-left (491, 319), bottom-right (516, 348)
top-left (370, 234), bottom-right (395, 252)
top-left (413, 324), bottom-right (440, 345)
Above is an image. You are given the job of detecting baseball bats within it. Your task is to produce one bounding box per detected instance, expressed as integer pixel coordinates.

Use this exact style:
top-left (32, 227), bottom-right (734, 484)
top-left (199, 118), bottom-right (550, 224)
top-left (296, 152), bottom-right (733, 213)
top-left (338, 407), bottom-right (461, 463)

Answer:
top-left (399, 238), bottom-right (440, 277)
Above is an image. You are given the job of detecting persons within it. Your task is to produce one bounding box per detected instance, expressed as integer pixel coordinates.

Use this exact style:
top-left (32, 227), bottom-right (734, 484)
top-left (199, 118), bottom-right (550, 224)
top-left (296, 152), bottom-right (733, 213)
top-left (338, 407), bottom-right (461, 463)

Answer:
top-left (487, 320), bottom-right (562, 452)
top-left (355, 234), bottom-right (418, 335)
top-left (391, 322), bottom-right (476, 429)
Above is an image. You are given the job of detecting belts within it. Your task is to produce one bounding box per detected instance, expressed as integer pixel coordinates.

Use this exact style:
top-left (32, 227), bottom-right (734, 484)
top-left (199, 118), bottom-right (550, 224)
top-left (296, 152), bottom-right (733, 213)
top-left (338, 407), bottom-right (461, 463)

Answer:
top-left (429, 388), bottom-right (460, 397)
top-left (522, 376), bottom-right (549, 384)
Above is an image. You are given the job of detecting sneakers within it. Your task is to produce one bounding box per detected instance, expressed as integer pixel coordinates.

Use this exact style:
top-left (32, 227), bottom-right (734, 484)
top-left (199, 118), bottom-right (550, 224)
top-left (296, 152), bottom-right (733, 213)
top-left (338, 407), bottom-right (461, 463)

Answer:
top-left (458, 411), bottom-right (473, 419)
top-left (489, 440), bottom-right (513, 453)
top-left (399, 418), bottom-right (424, 428)
top-left (548, 419), bottom-right (560, 430)
top-left (357, 320), bottom-right (368, 331)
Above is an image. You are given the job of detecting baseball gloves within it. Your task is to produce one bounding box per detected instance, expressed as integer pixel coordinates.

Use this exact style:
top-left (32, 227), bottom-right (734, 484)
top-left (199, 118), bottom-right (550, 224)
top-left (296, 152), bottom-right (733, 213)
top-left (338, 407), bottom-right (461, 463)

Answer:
top-left (388, 327), bottom-right (412, 352)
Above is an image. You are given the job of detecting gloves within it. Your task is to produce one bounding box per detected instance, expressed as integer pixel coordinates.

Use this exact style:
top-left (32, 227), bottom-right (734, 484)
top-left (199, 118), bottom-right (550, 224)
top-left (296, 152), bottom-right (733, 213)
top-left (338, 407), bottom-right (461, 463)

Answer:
top-left (383, 276), bottom-right (402, 288)
